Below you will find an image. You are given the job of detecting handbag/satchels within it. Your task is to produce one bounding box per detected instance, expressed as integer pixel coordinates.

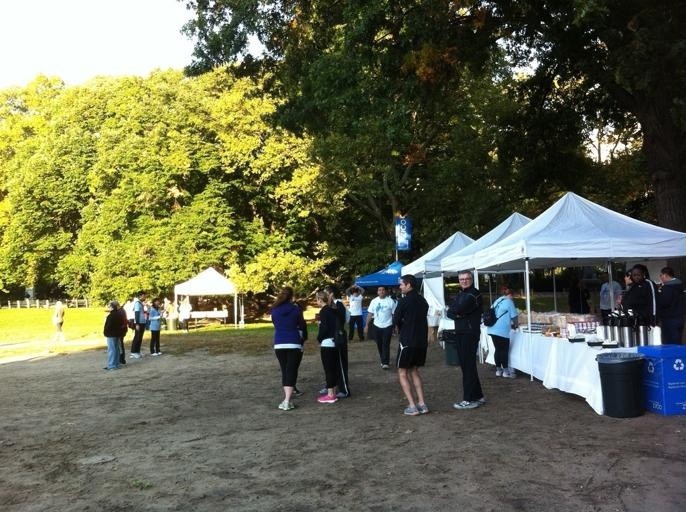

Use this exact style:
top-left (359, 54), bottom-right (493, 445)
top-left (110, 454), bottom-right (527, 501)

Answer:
top-left (127, 318), bottom-right (136, 330)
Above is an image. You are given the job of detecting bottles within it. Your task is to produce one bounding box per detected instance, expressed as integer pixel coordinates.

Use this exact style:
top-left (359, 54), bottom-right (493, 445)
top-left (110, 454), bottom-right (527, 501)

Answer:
top-left (604, 311), bottom-right (647, 347)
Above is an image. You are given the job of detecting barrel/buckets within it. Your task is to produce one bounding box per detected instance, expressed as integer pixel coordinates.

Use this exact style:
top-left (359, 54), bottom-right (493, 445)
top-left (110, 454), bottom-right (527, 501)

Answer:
top-left (442, 329), bottom-right (460, 365)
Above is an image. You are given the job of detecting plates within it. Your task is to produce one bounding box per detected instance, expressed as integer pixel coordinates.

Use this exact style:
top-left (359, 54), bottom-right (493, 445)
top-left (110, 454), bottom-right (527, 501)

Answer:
top-left (569, 338), bottom-right (618, 348)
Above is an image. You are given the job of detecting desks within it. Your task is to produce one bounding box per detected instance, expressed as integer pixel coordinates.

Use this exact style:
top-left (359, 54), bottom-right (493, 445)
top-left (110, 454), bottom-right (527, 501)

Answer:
top-left (436, 316), bottom-right (637, 416)
top-left (189, 309), bottom-right (229, 328)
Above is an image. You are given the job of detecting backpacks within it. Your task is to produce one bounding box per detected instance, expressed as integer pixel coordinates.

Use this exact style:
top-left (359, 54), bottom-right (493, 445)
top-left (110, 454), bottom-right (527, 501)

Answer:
top-left (482, 298), bottom-right (508, 326)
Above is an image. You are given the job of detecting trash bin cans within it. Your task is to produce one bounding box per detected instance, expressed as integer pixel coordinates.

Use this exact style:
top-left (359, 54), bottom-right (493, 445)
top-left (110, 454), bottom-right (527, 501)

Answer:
top-left (638, 344), bottom-right (686, 416)
top-left (166, 317), bottom-right (177, 332)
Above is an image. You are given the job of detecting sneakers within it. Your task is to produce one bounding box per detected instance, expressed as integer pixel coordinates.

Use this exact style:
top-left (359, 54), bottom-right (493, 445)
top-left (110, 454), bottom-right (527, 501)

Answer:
top-left (404, 405), bottom-right (420, 416)
top-left (130, 351), bottom-right (162, 360)
top-left (478, 398), bottom-right (486, 404)
top-left (417, 403), bottom-right (429, 414)
top-left (495, 370), bottom-right (502, 376)
top-left (503, 370), bottom-right (516, 378)
top-left (278, 385), bottom-right (348, 411)
top-left (453, 400), bottom-right (479, 409)
top-left (383, 364), bottom-right (390, 369)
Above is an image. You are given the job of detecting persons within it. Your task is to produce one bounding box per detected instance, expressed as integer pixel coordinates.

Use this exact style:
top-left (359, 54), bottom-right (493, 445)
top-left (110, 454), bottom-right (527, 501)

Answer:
top-left (270, 286), bottom-right (307, 410)
top-left (52, 299), bottom-right (66, 344)
top-left (484, 283), bottom-right (520, 380)
top-left (444, 270), bottom-right (487, 410)
top-left (599, 264), bottom-right (686, 345)
top-left (101, 290), bottom-right (191, 371)
top-left (568, 275), bottom-right (592, 314)
top-left (290, 280), bottom-right (402, 405)
top-left (388, 275), bottom-right (431, 416)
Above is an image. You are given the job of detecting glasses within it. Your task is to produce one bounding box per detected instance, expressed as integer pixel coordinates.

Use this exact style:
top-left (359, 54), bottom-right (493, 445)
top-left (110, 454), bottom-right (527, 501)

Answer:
top-left (459, 278), bottom-right (471, 280)
